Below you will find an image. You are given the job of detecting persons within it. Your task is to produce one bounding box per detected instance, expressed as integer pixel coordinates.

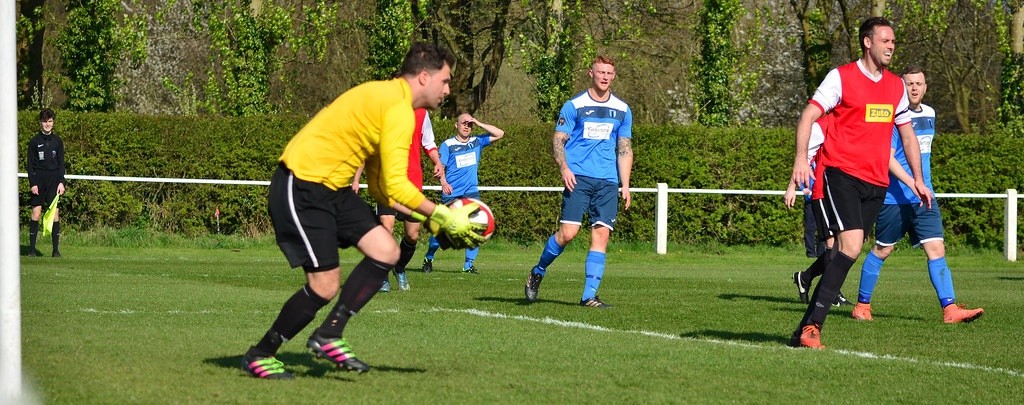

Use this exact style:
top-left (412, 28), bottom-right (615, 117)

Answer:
top-left (352, 107), bottom-right (444, 292)
top-left (792, 17), bottom-right (934, 348)
top-left (785, 123), bottom-right (855, 306)
top-left (851, 65), bottom-right (984, 324)
top-left (525, 55), bottom-right (633, 306)
top-left (420, 113), bottom-right (504, 274)
top-left (242, 40), bottom-right (488, 380)
top-left (27, 107), bottom-right (68, 257)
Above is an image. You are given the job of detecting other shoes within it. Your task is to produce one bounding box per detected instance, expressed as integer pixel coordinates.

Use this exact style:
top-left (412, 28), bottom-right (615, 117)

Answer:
top-left (53, 251), bottom-right (60, 257)
top-left (29, 253), bottom-right (36, 257)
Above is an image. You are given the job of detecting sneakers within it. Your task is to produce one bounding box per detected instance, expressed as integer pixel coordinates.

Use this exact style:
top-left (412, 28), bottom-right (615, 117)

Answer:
top-left (792, 271), bottom-right (811, 304)
top-left (580, 295), bottom-right (613, 309)
top-left (380, 281), bottom-right (391, 292)
top-left (853, 304), bottom-right (873, 321)
top-left (306, 327), bottom-right (370, 374)
top-left (832, 292), bottom-right (852, 306)
top-left (525, 266), bottom-right (543, 301)
top-left (791, 323), bottom-right (825, 350)
top-left (463, 266), bottom-right (479, 274)
top-left (393, 268), bottom-right (411, 291)
top-left (943, 304), bottom-right (984, 324)
top-left (241, 346), bottom-right (294, 381)
top-left (423, 257), bottom-right (434, 273)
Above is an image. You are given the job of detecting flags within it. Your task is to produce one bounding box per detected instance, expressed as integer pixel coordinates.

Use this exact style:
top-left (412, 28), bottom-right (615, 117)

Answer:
top-left (43, 194), bottom-right (59, 236)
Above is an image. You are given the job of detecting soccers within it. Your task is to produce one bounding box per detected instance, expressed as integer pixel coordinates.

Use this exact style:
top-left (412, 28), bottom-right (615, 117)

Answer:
top-left (447, 196), bottom-right (494, 242)
